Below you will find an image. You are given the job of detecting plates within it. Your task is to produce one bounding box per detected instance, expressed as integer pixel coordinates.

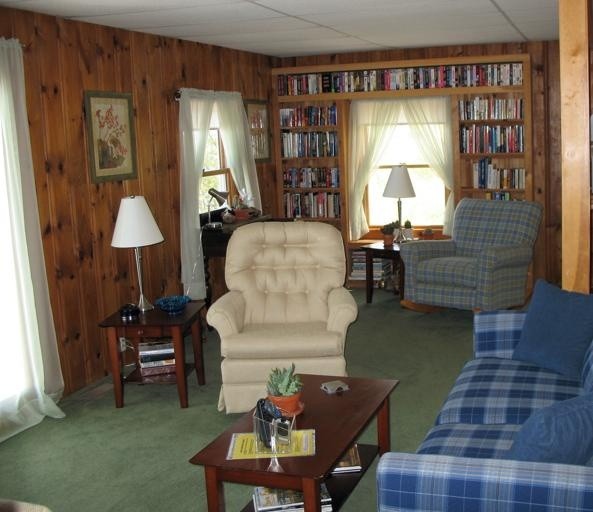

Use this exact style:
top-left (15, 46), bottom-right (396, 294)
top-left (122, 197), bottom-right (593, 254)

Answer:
top-left (154, 295), bottom-right (191, 314)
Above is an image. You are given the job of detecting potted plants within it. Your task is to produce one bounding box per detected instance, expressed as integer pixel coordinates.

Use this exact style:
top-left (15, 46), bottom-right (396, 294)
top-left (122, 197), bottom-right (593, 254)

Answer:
top-left (417, 228), bottom-right (434, 240)
top-left (263, 361), bottom-right (305, 418)
top-left (379, 224), bottom-right (395, 247)
top-left (391, 220), bottom-right (399, 239)
top-left (402, 219), bottom-right (413, 239)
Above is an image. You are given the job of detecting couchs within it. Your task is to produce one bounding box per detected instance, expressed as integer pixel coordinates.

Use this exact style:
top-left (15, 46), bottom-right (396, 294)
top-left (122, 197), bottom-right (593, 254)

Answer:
top-left (399, 196), bottom-right (544, 313)
top-left (374, 309), bottom-right (593, 512)
top-left (204, 221), bottom-right (358, 415)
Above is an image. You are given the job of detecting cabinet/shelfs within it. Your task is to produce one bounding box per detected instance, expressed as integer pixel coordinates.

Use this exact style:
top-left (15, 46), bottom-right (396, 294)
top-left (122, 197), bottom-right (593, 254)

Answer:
top-left (270, 52), bottom-right (535, 289)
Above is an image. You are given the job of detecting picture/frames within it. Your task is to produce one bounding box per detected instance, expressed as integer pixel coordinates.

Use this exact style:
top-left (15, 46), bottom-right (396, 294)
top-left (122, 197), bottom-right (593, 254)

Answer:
top-left (81, 89), bottom-right (139, 186)
top-left (243, 98), bottom-right (272, 164)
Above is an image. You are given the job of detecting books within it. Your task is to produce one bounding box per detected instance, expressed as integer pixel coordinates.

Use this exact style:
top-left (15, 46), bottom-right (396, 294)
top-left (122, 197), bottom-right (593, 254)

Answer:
top-left (350, 250), bottom-right (396, 280)
top-left (280, 132), bottom-right (338, 159)
top-left (456, 97), bottom-right (523, 121)
top-left (333, 442), bottom-right (361, 473)
top-left (277, 63), bottom-right (524, 97)
top-left (135, 340), bottom-right (178, 385)
top-left (459, 124), bottom-right (525, 154)
top-left (462, 156), bottom-right (526, 191)
top-left (283, 191), bottom-right (341, 221)
top-left (281, 165), bottom-right (339, 189)
top-left (483, 192), bottom-right (522, 201)
top-left (277, 103), bottom-right (336, 128)
top-left (253, 481), bottom-right (332, 512)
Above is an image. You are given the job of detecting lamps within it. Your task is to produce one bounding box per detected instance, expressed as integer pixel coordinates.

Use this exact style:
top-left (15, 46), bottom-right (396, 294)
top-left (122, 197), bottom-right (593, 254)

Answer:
top-left (108, 194), bottom-right (165, 312)
top-left (203, 187), bottom-right (230, 229)
top-left (382, 165), bottom-right (416, 244)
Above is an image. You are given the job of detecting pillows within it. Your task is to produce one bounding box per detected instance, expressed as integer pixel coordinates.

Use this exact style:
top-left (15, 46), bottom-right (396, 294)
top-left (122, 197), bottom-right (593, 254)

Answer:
top-left (511, 278), bottom-right (593, 380)
top-left (502, 392), bottom-right (593, 467)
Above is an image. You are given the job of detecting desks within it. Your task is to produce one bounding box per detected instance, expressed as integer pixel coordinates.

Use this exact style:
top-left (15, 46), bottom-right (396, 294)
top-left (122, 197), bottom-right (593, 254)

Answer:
top-left (201, 217), bottom-right (294, 312)
